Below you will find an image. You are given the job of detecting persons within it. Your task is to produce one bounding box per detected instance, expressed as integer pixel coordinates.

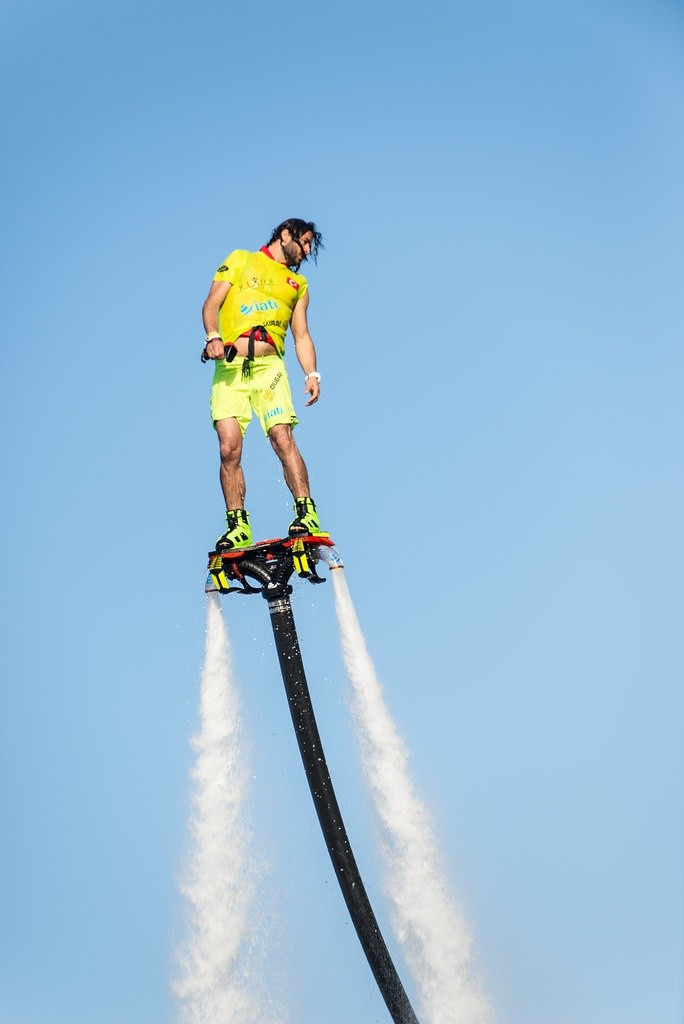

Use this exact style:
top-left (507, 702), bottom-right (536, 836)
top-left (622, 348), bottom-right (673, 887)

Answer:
top-left (201, 218), bottom-right (321, 551)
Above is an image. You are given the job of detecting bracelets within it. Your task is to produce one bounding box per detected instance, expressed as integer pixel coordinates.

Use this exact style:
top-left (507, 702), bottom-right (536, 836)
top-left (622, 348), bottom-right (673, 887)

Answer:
top-left (306, 372), bottom-right (321, 382)
top-left (205, 332), bottom-right (222, 345)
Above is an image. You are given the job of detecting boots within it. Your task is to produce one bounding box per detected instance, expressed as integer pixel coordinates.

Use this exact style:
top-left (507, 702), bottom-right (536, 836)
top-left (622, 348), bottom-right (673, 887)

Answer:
top-left (215, 509), bottom-right (252, 550)
top-left (289, 497), bottom-right (319, 535)
